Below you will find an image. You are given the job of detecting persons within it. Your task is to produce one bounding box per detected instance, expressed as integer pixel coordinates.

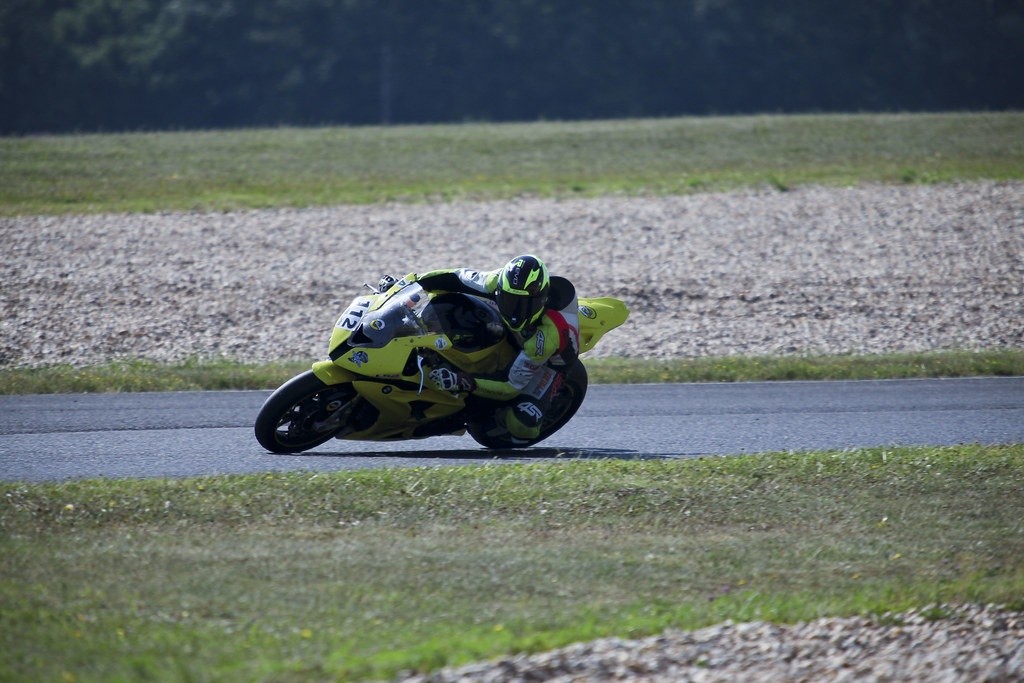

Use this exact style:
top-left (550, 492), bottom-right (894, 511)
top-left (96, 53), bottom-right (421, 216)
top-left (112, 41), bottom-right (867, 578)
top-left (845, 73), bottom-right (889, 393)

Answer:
top-left (378, 255), bottom-right (580, 450)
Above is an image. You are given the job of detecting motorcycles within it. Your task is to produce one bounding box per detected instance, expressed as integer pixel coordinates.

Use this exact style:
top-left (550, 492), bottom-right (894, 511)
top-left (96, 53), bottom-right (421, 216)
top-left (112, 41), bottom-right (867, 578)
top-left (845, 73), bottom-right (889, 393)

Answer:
top-left (254, 271), bottom-right (630, 455)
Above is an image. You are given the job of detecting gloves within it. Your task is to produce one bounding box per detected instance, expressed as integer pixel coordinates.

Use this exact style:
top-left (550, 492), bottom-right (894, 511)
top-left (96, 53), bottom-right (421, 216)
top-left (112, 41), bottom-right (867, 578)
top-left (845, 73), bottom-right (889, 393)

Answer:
top-left (429, 368), bottom-right (477, 393)
top-left (378, 274), bottom-right (399, 292)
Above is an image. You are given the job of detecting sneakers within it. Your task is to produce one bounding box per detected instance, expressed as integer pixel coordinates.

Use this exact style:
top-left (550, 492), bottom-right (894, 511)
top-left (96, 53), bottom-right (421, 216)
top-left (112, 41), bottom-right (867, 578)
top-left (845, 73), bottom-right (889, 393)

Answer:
top-left (484, 410), bottom-right (510, 437)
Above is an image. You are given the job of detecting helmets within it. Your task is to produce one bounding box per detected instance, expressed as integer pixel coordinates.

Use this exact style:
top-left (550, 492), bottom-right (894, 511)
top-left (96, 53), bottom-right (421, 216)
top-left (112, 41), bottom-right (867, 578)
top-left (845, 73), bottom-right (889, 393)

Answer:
top-left (496, 254), bottom-right (550, 332)
